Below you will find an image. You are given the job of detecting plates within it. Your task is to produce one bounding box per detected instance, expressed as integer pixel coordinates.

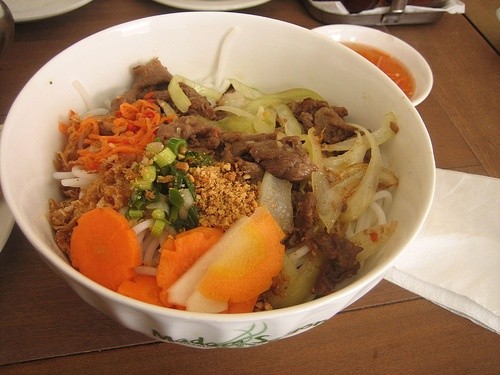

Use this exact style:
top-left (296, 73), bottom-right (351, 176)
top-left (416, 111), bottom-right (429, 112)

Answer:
top-left (0, 124), bottom-right (15, 253)
top-left (1, 0), bottom-right (92, 23)
top-left (153, 0), bottom-right (271, 11)
top-left (310, 23), bottom-right (434, 106)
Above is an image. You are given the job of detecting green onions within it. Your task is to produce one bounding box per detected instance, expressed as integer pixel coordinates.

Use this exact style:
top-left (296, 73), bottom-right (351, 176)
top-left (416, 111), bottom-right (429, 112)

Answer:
top-left (124, 138), bottom-right (217, 233)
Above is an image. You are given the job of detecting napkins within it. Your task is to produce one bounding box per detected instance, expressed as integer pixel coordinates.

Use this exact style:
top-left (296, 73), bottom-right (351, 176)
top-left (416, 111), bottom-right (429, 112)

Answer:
top-left (383, 168), bottom-right (500, 335)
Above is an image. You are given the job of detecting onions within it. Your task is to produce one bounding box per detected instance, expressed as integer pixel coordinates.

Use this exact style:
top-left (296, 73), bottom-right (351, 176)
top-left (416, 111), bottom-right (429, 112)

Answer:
top-left (169, 77), bottom-right (399, 309)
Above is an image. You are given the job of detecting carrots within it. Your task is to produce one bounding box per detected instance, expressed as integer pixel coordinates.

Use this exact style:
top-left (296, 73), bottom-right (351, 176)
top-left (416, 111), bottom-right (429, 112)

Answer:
top-left (69, 206), bottom-right (286, 314)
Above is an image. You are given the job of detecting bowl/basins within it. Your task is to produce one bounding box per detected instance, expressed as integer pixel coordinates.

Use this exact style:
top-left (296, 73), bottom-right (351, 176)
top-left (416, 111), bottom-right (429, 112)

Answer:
top-left (0, 11), bottom-right (436, 349)
